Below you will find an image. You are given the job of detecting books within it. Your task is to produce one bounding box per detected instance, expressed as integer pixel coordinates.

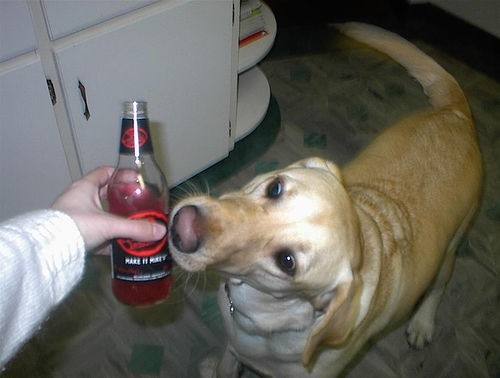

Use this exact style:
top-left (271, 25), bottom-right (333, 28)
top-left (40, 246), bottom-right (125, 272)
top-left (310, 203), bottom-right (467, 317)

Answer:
top-left (239, 0), bottom-right (268, 49)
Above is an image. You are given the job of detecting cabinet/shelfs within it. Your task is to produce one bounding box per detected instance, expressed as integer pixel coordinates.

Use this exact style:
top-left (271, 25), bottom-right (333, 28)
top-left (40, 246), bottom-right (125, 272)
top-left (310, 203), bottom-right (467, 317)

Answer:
top-left (229, 0), bottom-right (276, 159)
top-left (0, 1), bottom-right (228, 227)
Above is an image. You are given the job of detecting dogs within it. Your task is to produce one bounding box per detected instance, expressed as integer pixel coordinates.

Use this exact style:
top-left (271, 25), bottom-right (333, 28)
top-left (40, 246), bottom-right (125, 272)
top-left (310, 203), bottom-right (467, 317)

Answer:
top-left (168, 20), bottom-right (482, 378)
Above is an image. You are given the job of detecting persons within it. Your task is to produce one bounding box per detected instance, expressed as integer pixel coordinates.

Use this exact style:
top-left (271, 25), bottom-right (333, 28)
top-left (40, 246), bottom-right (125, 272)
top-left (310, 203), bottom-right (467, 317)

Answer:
top-left (0, 164), bottom-right (168, 378)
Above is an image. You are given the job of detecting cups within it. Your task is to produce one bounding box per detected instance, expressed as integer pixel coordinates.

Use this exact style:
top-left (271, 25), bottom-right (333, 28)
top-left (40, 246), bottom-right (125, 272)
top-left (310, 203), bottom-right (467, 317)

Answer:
top-left (105, 98), bottom-right (172, 310)
